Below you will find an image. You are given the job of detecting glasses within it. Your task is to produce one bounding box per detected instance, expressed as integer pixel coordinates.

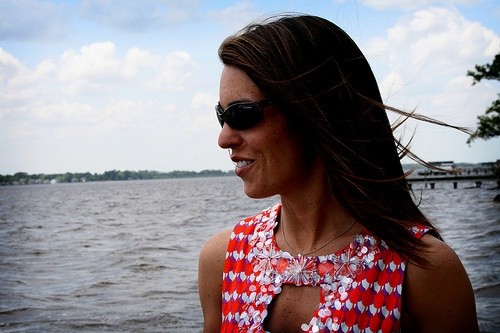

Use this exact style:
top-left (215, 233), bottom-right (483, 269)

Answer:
top-left (215, 99), bottom-right (272, 131)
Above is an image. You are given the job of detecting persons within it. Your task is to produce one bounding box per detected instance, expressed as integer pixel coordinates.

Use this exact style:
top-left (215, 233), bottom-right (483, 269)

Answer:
top-left (197, 14), bottom-right (481, 333)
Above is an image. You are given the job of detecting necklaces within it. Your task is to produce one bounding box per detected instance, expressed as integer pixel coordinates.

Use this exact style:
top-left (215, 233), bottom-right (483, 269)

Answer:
top-left (280, 204), bottom-right (359, 255)
